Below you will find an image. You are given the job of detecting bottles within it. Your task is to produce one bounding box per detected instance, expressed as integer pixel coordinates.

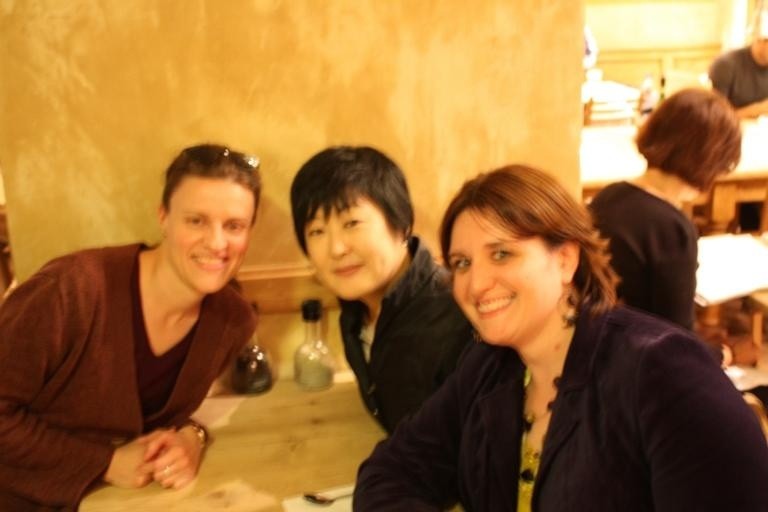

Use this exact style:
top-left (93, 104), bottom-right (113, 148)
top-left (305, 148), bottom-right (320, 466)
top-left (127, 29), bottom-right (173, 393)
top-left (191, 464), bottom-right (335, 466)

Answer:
top-left (289, 299), bottom-right (335, 391)
top-left (229, 299), bottom-right (276, 392)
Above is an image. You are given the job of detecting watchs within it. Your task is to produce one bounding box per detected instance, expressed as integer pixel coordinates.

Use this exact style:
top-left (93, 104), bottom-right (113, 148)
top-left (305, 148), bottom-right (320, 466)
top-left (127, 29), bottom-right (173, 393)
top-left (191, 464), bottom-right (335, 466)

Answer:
top-left (176, 418), bottom-right (208, 445)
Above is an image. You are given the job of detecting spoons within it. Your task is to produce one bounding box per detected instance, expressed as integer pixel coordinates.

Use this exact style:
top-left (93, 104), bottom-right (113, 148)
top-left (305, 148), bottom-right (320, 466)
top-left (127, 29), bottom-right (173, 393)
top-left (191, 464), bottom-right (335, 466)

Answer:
top-left (303, 486), bottom-right (353, 504)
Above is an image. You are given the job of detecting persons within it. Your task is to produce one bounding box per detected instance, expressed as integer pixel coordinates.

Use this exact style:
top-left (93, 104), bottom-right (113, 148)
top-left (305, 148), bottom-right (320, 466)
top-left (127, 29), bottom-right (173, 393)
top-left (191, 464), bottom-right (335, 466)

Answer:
top-left (352, 165), bottom-right (768, 510)
top-left (288, 145), bottom-right (481, 437)
top-left (585, 90), bottom-right (768, 418)
top-left (708, 35), bottom-right (768, 121)
top-left (0, 143), bottom-right (265, 512)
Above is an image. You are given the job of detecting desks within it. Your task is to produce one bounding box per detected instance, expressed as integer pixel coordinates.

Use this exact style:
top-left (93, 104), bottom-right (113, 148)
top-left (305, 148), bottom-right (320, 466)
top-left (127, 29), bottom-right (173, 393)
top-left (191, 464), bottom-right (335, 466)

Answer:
top-left (577, 96), bottom-right (768, 374)
top-left (73, 355), bottom-right (391, 512)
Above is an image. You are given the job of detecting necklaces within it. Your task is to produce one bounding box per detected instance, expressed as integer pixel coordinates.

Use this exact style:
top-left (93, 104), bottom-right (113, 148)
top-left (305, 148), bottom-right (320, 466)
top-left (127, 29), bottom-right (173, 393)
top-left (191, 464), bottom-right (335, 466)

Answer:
top-left (519, 360), bottom-right (562, 484)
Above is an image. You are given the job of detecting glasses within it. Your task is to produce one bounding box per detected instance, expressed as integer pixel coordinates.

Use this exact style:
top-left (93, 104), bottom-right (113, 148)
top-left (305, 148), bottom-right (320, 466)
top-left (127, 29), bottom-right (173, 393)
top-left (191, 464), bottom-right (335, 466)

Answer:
top-left (180, 146), bottom-right (259, 172)
